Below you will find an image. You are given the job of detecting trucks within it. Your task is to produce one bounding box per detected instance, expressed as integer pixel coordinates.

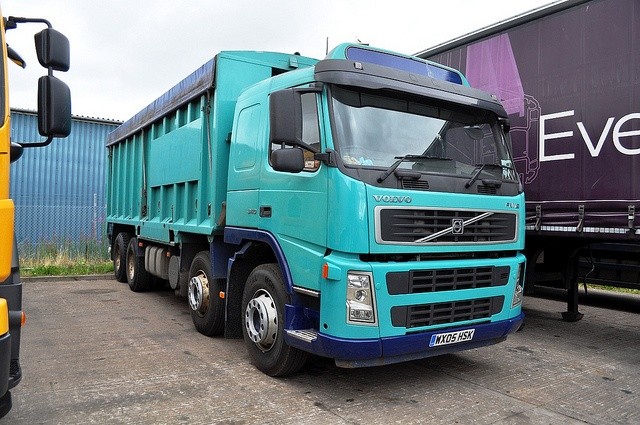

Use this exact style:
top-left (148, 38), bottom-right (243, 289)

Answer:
top-left (0, 4), bottom-right (71, 418)
top-left (103, 41), bottom-right (529, 382)
